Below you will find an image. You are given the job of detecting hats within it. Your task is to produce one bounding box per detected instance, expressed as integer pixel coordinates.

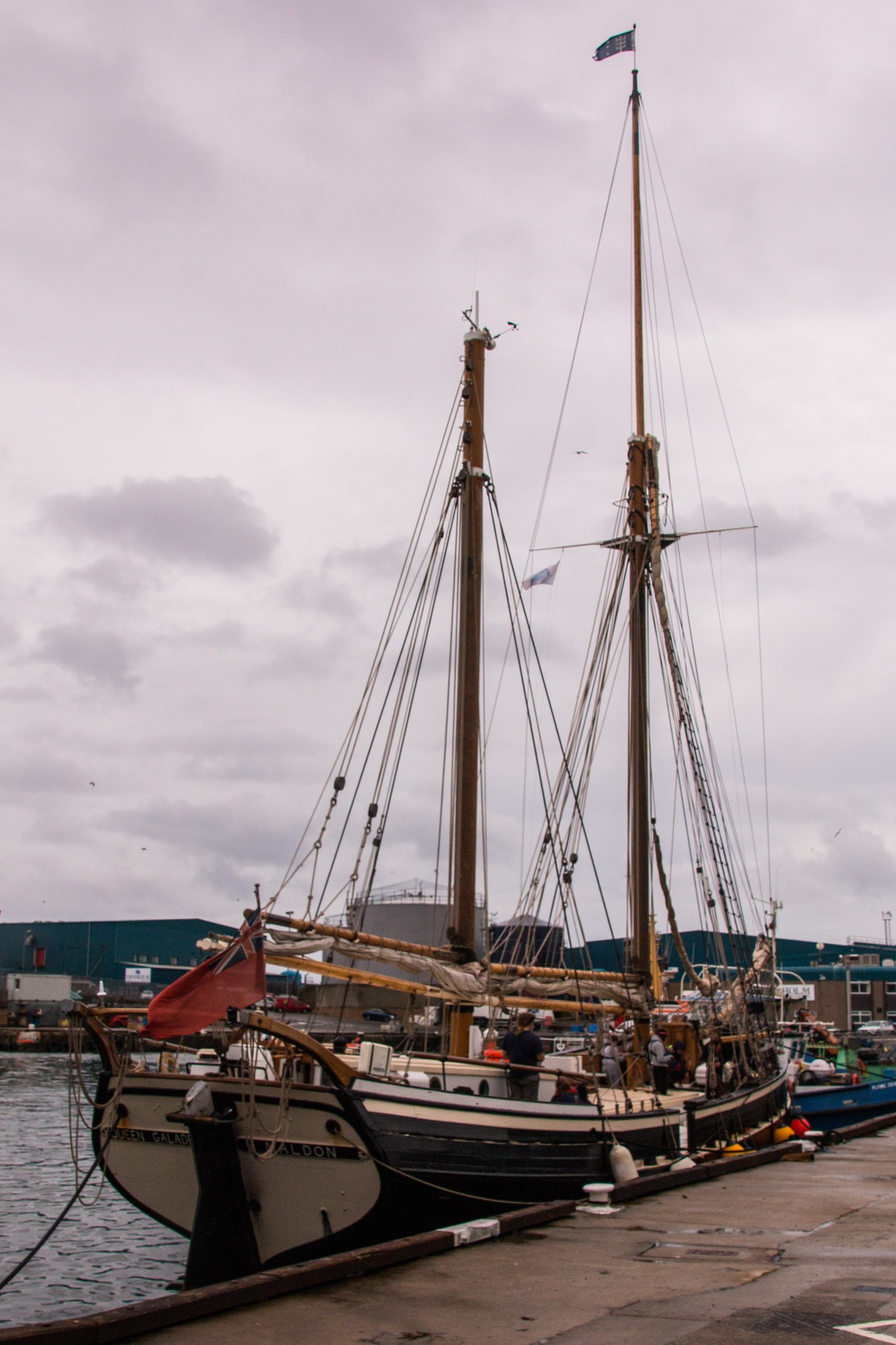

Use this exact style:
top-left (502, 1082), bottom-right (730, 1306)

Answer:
top-left (656, 1028), bottom-right (668, 1037)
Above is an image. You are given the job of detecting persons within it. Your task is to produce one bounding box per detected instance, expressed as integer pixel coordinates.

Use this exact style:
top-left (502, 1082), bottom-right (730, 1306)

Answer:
top-left (575, 1083), bottom-right (594, 1106)
top-left (668, 1040), bottom-right (689, 1084)
top-left (501, 1012), bottom-right (546, 1101)
top-left (647, 1028), bottom-right (676, 1094)
top-left (551, 1076), bottom-right (576, 1105)
top-left (599, 1033), bottom-right (624, 1087)
top-left (701, 1032), bottom-right (738, 1093)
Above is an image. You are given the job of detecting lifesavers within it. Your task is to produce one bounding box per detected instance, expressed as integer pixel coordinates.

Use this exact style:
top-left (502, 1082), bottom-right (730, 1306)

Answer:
top-left (482, 1049), bottom-right (510, 1063)
top-left (852, 1073), bottom-right (861, 1085)
top-left (799, 1069), bottom-right (817, 1085)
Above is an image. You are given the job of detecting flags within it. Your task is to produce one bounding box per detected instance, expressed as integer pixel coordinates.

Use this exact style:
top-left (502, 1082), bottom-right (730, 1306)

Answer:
top-left (137, 911), bottom-right (264, 1038)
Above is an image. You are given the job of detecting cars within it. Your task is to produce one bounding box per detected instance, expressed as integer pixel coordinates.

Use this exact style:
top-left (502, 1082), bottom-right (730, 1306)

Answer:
top-left (856, 1019), bottom-right (895, 1036)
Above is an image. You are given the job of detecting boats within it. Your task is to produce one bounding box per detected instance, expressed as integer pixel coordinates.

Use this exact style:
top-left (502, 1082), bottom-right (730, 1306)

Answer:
top-left (785, 1030), bottom-right (896, 1147)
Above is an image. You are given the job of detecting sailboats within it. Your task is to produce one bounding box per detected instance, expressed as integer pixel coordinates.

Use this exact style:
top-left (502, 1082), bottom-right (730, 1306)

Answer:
top-left (58, 26), bottom-right (811, 1299)
